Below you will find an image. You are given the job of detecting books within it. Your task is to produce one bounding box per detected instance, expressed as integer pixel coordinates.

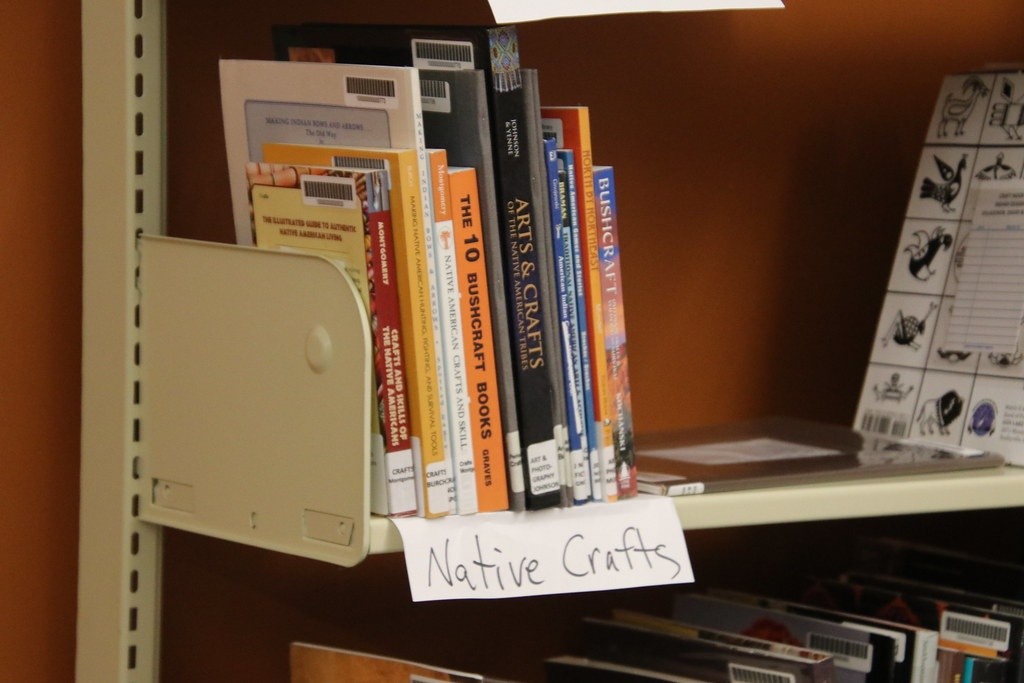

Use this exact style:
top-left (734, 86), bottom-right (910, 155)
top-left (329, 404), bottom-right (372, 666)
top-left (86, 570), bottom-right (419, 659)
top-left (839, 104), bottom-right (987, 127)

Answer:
top-left (219, 23), bottom-right (638, 514)
top-left (289, 539), bottom-right (1024, 683)
top-left (630, 418), bottom-right (1004, 496)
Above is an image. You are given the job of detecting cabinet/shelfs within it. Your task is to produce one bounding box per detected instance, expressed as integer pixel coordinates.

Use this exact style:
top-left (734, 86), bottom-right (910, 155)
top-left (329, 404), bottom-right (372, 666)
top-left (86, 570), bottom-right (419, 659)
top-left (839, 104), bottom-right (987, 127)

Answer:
top-left (81, 0), bottom-right (1024, 682)
